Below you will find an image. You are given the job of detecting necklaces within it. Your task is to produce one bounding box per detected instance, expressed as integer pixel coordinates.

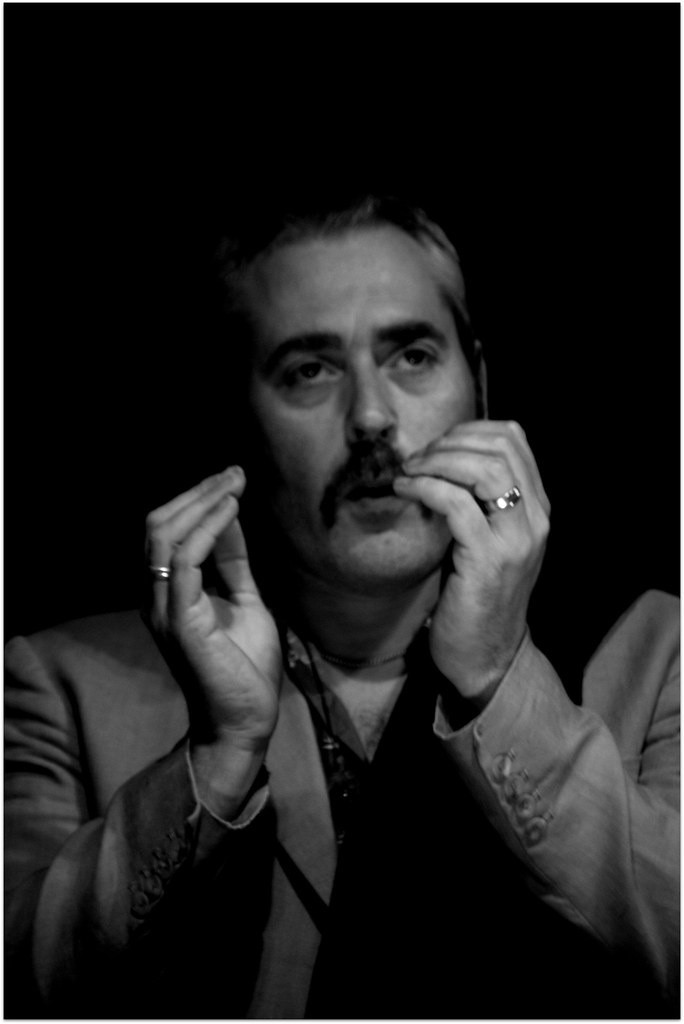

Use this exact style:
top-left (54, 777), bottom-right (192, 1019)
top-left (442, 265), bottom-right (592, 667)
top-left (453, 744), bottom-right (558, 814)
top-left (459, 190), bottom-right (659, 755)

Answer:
top-left (321, 647), bottom-right (409, 669)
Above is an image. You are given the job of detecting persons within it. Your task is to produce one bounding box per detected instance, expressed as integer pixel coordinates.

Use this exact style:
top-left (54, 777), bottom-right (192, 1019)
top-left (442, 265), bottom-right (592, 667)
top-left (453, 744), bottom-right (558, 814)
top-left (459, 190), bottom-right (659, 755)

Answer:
top-left (4, 193), bottom-right (680, 1019)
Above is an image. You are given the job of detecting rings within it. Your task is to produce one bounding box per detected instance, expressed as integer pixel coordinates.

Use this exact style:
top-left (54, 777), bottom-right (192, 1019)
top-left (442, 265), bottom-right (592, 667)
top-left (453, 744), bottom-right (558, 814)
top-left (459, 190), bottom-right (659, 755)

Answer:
top-left (484, 485), bottom-right (522, 515)
top-left (149, 565), bottom-right (171, 579)
